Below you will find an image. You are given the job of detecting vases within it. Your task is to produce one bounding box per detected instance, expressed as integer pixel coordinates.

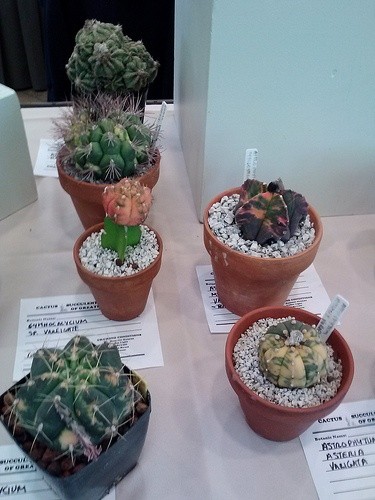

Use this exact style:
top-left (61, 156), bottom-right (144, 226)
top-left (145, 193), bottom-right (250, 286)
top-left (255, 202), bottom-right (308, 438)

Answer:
top-left (74, 221), bottom-right (162, 321)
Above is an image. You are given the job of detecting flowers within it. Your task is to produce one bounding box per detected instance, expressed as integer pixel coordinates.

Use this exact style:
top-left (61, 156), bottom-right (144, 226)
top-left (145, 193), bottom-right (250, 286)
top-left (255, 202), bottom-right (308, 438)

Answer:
top-left (100, 178), bottom-right (154, 261)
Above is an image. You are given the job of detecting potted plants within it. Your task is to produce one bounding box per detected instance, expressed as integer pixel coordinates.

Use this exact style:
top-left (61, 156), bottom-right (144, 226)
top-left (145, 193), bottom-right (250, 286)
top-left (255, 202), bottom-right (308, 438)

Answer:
top-left (57, 94), bottom-right (160, 227)
top-left (65, 20), bottom-right (160, 127)
top-left (0, 335), bottom-right (152, 500)
top-left (203, 180), bottom-right (322, 316)
top-left (226, 305), bottom-right (355, 442)
